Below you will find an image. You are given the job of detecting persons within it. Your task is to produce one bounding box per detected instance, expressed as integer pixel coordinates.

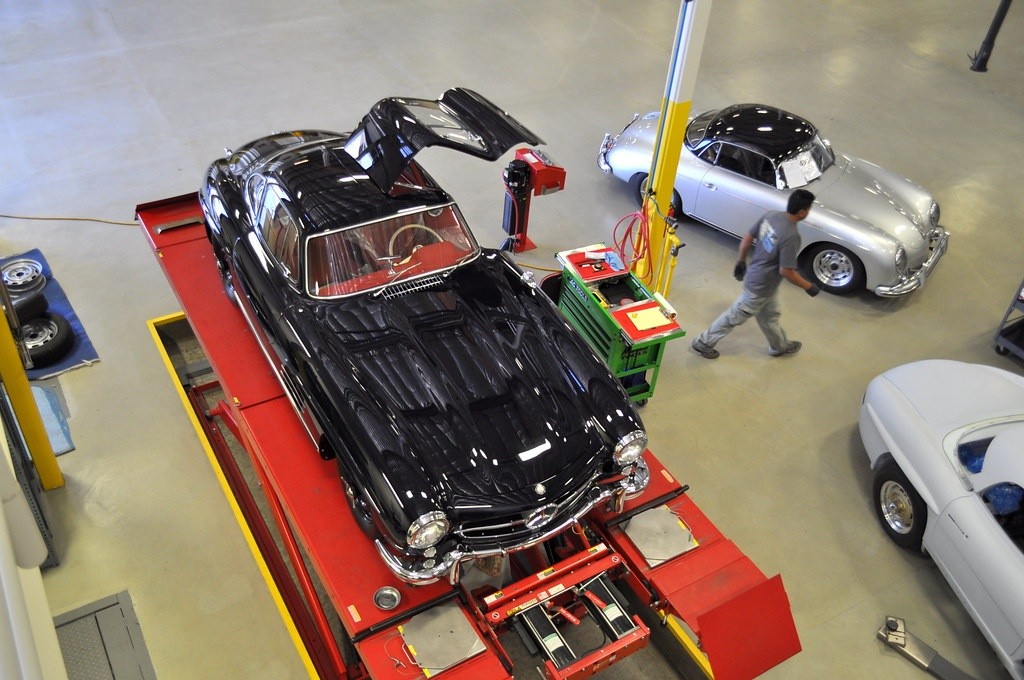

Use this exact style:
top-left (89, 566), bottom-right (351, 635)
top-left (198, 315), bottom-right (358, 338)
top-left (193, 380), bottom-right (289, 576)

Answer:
top-left (691, 190), bottom-right (820, 358)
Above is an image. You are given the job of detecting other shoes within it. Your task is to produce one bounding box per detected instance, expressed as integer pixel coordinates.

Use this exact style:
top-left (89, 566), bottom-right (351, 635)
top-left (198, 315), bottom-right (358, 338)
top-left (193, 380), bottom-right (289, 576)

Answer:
top-left (692, 338), bottom-right (720, 359)
top-left (769, 341), bottom-right (802, 357)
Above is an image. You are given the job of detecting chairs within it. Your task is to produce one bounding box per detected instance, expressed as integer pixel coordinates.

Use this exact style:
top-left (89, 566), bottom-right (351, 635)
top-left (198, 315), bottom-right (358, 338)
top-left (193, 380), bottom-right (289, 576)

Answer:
top-left (275, 220), bottom-right (359, 289)
top-left (357, 214), bottom-right (429, 272)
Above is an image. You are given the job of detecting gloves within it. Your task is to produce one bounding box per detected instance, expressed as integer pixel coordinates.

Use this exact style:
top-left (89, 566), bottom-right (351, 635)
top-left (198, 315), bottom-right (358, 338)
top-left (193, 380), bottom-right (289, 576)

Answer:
top-left (806, 285), bottom-right (819, 299)
top-left (733, 261), bottom-right (746, 283)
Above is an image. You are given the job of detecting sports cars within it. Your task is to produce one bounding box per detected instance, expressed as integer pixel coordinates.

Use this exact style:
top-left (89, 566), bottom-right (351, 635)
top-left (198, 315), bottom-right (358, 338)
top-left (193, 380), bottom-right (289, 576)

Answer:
top-left (858, 359), bottom-right (1024, 680)
top-left (596, 103), bottom-right (951, 299)
top-left (197, 88), bottom-right (649, 586)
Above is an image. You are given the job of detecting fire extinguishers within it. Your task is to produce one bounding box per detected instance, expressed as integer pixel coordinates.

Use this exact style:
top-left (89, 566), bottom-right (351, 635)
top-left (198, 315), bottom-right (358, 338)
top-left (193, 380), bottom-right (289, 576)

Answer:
top-left (663, 201), bottom-right (676, 237)
